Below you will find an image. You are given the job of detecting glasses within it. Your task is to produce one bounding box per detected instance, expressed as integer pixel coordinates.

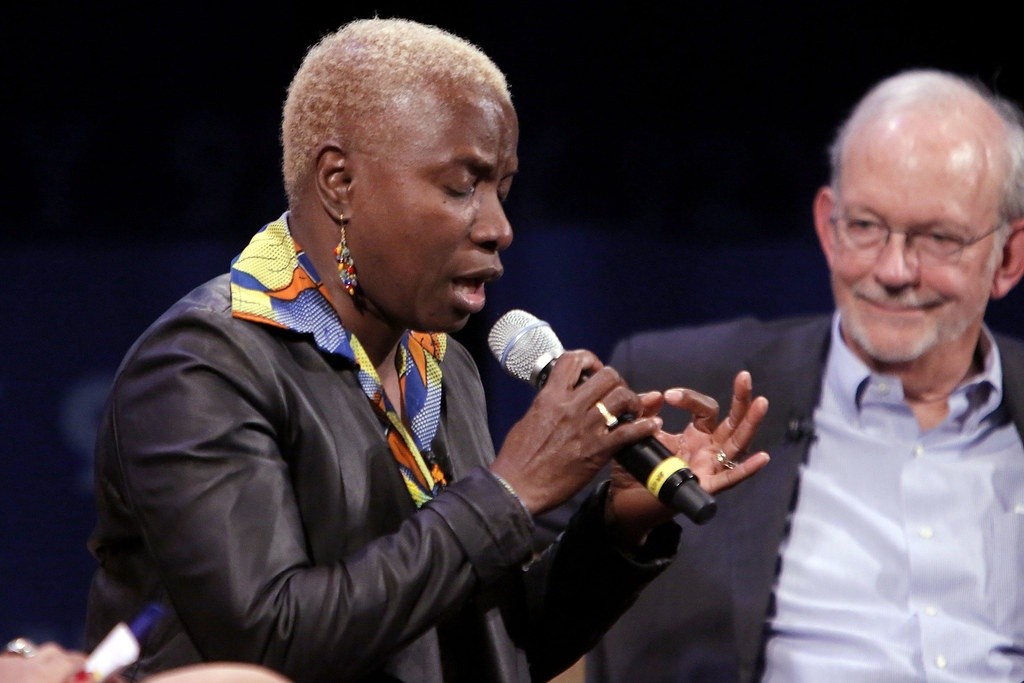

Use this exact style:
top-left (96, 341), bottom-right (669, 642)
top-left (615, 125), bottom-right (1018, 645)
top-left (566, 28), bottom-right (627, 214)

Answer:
top-left (826, 208), bottom-right (1006, 267)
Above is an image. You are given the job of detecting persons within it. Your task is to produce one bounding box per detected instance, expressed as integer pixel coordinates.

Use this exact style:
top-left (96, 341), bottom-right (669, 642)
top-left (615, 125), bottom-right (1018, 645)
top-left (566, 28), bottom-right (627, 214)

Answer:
top-left (502, 69), bottom-right (1024, 683)
top-left (85, 15), bottom-right (771, 683)
top-left (0, 637), bottom-right (294, 683)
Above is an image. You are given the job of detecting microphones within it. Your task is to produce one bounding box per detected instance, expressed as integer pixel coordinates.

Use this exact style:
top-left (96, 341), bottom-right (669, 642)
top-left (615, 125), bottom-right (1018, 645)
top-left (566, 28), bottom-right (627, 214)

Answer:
top-left (787, 416), bottom-right (805, 441)
top-left (489, 309), bottom-right (716, 524)
top-left (423, 450), bottom-right (436, 470)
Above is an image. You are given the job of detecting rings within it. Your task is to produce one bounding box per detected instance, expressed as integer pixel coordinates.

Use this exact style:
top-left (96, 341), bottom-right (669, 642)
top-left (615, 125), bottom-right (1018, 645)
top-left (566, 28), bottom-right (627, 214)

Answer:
top-left (6, 636), bottom-right (38, 658)
top-left (595, 401), bottom-right (618, 427)
top-left (717, 449), bottom-right (738, 470)
